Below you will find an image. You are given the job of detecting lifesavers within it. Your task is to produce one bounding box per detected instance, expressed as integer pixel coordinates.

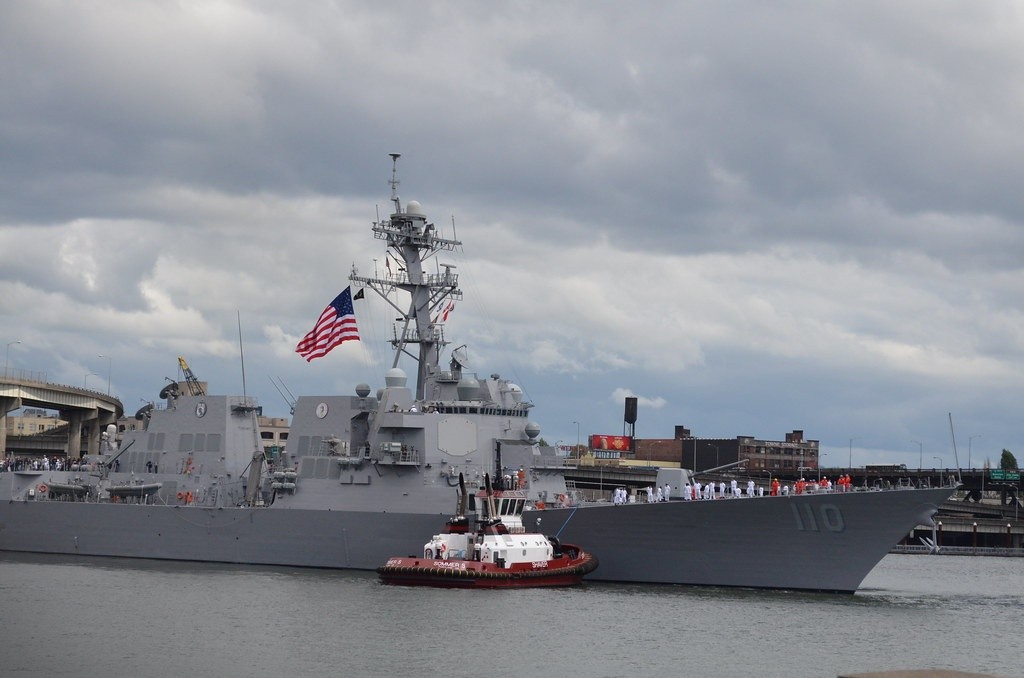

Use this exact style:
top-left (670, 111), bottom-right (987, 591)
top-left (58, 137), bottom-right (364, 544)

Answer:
top-left (177, 492), bottom-right (184, 499)
top-left (39, 484), bottom-right (48, 492)
top-left (560, 493), bottom-right (565, 502)
top-left (185, 491), bottom-right (192, 503)
top-left (114, 495), bottom-right (119, 502)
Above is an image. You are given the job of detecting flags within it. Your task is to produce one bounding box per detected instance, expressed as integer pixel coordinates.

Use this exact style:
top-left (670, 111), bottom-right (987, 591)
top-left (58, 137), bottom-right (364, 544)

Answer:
top-left (354, 288), bottom-right (364, 300)
top-left (295, 285), bottom-right (360, 362)
top-left (437, 300), bottom-right (454, 323)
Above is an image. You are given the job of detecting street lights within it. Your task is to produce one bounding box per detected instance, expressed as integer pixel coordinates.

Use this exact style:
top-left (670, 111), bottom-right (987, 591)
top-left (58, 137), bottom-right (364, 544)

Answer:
top-left (5, 340), bottom-right (21, 378)
top-left (99, 355), bottom-right (112, 396)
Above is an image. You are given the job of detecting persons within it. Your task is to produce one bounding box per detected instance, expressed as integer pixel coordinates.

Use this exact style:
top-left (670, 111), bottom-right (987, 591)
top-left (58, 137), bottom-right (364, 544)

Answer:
top-left (646, 486), bottom-right (654, 503)
top-left (771, 474), bottom-right (850, 496)
top-left (665, 484), bottom-right (670, 502)
top-left (614, 487), bottom-right (620, 505)
top-left (654, 486), bottom-right (662, 502)
top-left (517, 469), bottom-right (525, 487)
top-left (621, 487), bottom-right (627, 504)
top-left (0, 455), bottom-right (87, 472)
top-left (683, 479), bottom-right (755, 501)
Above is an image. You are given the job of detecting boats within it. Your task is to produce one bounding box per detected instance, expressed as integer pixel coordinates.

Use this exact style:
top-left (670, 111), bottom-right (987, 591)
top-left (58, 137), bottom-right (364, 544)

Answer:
top-left (375, 471), bottom-right (600, 590)
top-left (0, 154), bottom-right (966, 596)
top-left (818, 413), bottom-right (1024, 559)
